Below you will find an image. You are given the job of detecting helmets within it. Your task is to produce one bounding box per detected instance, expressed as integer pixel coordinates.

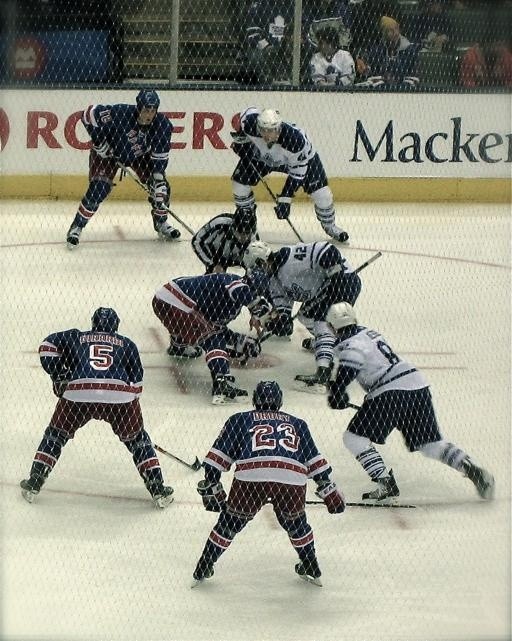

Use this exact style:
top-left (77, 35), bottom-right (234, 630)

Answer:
top-left (136, 89), bottom-right (161, 115)
top-left (248, 271), bottom-right (269, 297)
top-left (232, 206), bottom-right (256, 234)
top-left (91, 306), bottom-right (121, 332)
top-left (253, 379), bottom-right (283, 406)
top-left (243, 240), bottom-right (273, 269)
top-left (325, 301), bottom-right (357, 330)
top-left (256, 109), bottom-right (282, 142)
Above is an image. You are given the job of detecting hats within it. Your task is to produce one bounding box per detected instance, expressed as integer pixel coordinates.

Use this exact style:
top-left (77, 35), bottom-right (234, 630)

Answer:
top-left (377, 15), bottom-right (399, 32)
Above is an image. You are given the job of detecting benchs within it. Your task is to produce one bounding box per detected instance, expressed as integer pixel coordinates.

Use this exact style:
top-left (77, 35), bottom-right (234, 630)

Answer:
top-left (119, 5), bottom-right (245, 90)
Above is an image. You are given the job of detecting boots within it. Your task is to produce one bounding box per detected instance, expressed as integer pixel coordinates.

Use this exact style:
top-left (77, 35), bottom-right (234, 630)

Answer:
top-left (293, 366), bottom-right (331, 395)
top-left (301, 338), bottom-right (316, 351)
top-left (190, 550), bottom-right (218, 590)
top-left (19, 459), bottom-right (53, 504)
top-left (210, 375), bottom-right (250, 405)
top-left (152, 218), bottom-right (181, 243)
top-left (140, 464), bottom-right (175, 509)
top-left (294, 548), bottom-right (323, 589)
top-left (361, 467), bottom-right (401, 503)
top-left (320, 222), bottom-right (350, 245)
top-left (166, 340), bottom-right (204, 360)
top-left (460, 457), bottom-right (496, 500)
top-left (65, 223), bottom-right (83, 251)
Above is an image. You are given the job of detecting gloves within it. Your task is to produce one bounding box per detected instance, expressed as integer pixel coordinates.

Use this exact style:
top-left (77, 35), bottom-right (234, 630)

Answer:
top-left (197, 480), bottom-right (227, 513)
top-left (274, 198), bottom-right (291, 220)
top-left (328, 387), bottom-right (350, 412)
top-left (314, 481), bottom-right (346, 515)
top-left (93, 139), bottom-right (116, 160)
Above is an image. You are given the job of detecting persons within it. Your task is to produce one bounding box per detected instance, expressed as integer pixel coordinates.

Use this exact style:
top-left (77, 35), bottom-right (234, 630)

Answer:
top-left (65, 88), bottom-right (182, 244)
top-left (189, 206), bottom-right (262, 276)
top-left (243, 240), bottom-right (364, 390)
top-left (18, 304), bottom-right (176, 505)
top-left (229, 102), bottom-right (350, 244)
top-left (322, 299), bottom-right (497, 504)
top-left (193, 378), bottom-right (346, 581)
top-left (229, 0), bottom-right (512, 89)
top-left (150, 268), bottom-right (275, 402)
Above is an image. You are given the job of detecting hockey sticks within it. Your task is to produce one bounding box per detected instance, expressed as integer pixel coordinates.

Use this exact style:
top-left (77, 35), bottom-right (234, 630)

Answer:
top-left (238, 327), bottom-right (266, 369)
top-left (262, 500), bottom-right (415, 508)
top-left (154, 443), bottom-right (201, 470)
top-left (228, 252), bottom-right (383, 359)
